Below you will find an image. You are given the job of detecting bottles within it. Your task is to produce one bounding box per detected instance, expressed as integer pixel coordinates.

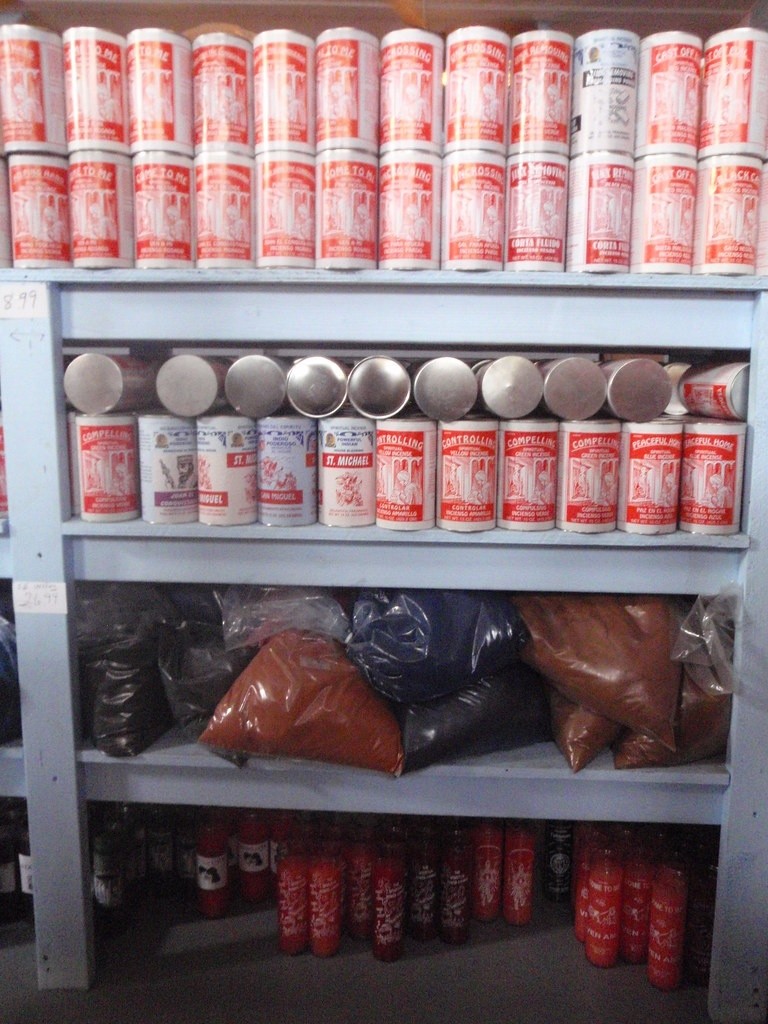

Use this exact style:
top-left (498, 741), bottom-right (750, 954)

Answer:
top-left (0, 795), bottom-right (692, 992)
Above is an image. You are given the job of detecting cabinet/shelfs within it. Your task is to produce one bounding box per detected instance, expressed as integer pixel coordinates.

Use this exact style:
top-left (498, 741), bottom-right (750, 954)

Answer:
top-left (0, 268), bottom-right (767, 1023)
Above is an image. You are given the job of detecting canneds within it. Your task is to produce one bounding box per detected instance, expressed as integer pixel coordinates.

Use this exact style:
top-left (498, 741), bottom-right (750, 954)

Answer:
top-left (0, 350), bottom-right (755, 535)
top-left (1, 23), bottom-right (768, 274)
